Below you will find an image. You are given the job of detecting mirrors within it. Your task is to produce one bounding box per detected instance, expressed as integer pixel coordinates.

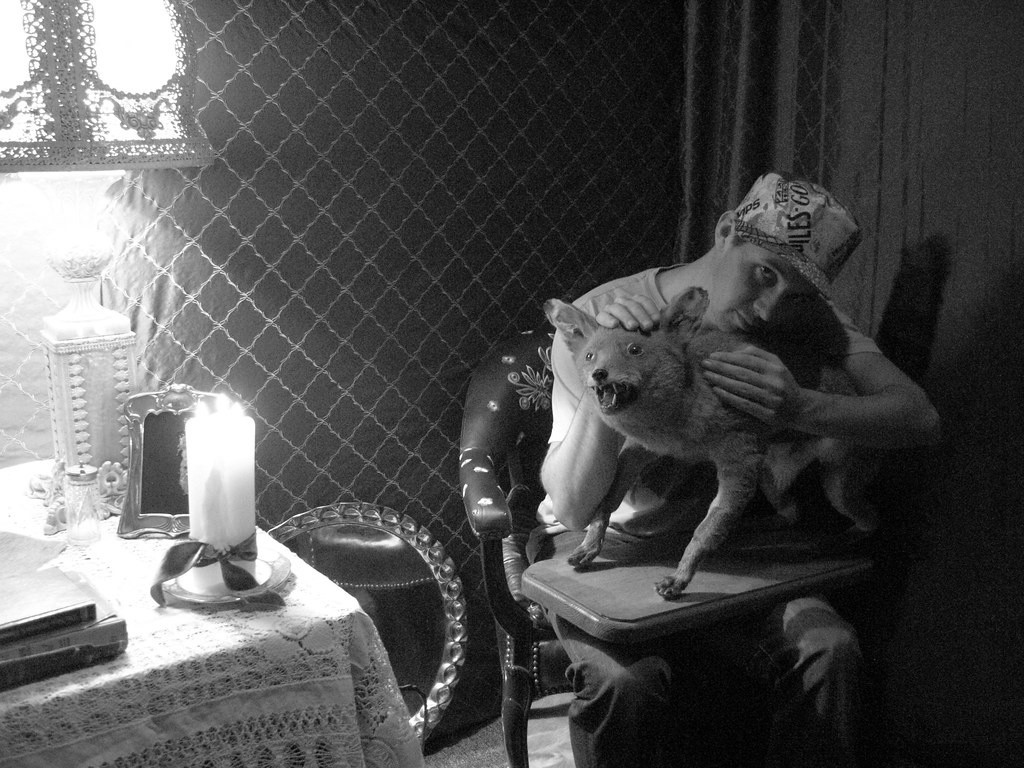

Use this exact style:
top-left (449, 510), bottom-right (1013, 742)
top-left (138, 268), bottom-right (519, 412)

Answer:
top-left (269, 503), bottom-right (469, 746)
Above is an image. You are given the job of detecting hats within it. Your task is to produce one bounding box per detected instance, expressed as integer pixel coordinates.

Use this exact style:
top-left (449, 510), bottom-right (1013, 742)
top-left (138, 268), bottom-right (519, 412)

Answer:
top-left (734, 170), bottom-right (861, 308)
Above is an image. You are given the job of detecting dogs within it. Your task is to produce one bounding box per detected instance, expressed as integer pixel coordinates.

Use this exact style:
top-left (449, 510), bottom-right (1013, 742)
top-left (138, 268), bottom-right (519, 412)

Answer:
top-left (544, 289), bottom-right (884, 598)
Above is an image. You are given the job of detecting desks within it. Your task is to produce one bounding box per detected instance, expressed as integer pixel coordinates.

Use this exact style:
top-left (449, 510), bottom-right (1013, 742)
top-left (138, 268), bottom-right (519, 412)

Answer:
top-left (0, 459), bottom-right (424, 768)
top-left (521, 536), bottom-right (877, 641)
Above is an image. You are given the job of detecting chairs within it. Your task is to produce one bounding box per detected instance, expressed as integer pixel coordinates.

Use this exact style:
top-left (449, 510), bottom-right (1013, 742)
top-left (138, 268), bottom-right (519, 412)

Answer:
top-left (457, 322), bottom-right (556, 768)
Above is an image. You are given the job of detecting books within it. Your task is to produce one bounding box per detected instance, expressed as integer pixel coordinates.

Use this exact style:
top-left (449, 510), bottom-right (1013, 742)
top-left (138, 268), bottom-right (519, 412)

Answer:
top-left (0, 566), bottom-right (128, 691)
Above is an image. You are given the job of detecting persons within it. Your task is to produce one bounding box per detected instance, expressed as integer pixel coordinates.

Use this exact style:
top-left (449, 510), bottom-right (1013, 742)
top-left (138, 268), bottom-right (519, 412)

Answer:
top-left (524, 174), bottom-right (941, 767)
